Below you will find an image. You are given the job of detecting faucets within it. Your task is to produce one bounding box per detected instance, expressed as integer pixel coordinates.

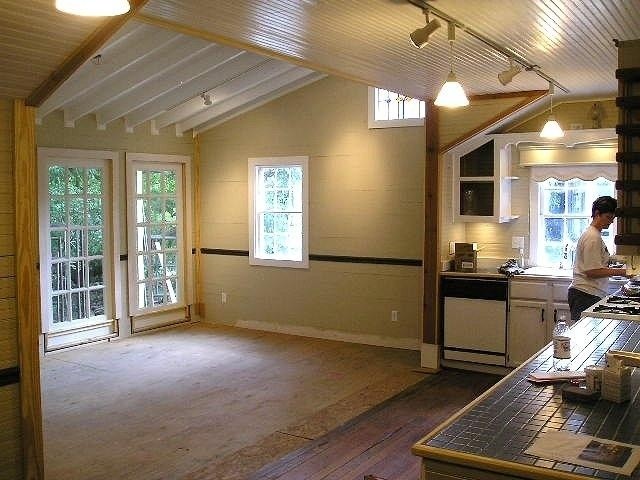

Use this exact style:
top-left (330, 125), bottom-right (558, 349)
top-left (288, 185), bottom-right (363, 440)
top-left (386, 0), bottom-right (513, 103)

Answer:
top-left (559, 262), bottom-right (563, 268)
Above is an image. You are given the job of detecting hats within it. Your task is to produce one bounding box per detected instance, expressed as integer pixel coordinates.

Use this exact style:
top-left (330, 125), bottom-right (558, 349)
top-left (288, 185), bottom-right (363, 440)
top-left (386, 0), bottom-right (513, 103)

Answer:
top-left (593, 196), bottom-right (616, 214)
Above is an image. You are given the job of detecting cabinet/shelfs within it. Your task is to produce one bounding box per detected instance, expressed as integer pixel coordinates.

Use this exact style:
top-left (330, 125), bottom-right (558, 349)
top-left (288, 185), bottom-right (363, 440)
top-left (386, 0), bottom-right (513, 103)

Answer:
top-left (450, 134), bottom-right (512, 225)
top-left (506, 280), bottom-right (573, 368)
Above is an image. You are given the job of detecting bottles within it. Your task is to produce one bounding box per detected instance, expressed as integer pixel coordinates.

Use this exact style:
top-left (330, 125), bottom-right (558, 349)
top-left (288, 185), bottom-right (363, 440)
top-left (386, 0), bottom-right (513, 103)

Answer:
top-left (519, 248), bottom-right (525, 268)
top-left (552, 316), bottom-right (571, 371)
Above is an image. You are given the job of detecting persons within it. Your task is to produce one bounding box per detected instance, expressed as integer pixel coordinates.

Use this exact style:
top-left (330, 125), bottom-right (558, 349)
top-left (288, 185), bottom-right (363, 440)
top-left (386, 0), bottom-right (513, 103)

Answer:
top-left (566, 195), bottom-right (635, 324)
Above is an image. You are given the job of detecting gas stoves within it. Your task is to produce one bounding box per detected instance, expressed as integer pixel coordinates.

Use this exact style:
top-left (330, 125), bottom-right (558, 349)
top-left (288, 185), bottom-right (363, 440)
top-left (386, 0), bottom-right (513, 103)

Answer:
top-left (581, 294), bottom-right (640, 321)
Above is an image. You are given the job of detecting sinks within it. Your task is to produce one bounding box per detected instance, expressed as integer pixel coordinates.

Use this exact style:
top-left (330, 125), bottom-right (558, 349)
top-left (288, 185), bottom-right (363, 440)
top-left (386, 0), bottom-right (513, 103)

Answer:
top-left (553, 269), bottom-right (573, 277)
top-left (520, 266), bottom-right (553, 276)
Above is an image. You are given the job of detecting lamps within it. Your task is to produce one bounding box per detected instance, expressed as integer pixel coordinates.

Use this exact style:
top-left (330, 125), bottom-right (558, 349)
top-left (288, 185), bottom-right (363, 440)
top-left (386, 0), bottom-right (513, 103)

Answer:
top-left (408, 7), bottom-right (566, 142)
